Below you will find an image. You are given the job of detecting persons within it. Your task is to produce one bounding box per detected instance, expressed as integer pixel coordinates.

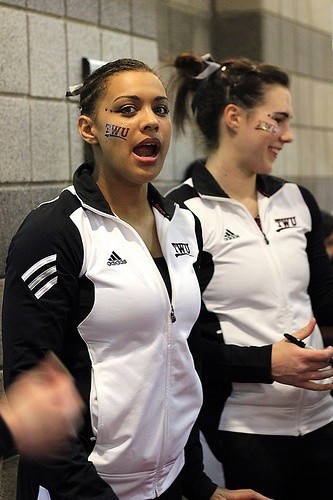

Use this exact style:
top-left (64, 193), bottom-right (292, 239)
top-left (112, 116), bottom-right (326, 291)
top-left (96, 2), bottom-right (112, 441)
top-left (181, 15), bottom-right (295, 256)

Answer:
top-left (160, 52), bottom-right (333, 500)
top-left (314, 207), bottom-right (333, 260)
top-left (3, 56), bottom-right (271, 500)
top-left (0, 353), bottom-right (87, 468)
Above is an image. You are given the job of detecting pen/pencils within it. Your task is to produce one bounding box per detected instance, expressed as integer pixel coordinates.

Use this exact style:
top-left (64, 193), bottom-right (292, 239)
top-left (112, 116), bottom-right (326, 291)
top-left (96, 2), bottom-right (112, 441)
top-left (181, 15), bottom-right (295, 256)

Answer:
top-left (284, 333), bottom-right (317, 351)
